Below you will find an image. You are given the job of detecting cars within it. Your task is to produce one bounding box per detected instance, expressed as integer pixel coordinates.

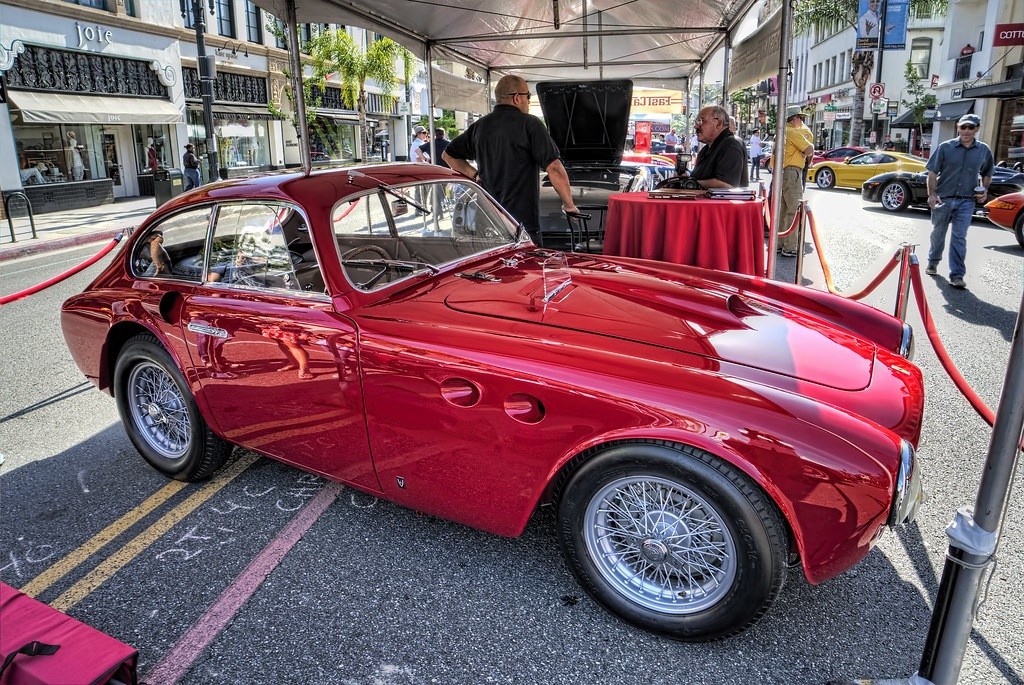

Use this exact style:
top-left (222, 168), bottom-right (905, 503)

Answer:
top-left (449, 76), bottom-right (696, 253)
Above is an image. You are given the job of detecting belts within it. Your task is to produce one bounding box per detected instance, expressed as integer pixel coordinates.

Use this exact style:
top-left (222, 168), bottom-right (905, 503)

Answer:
top-left (940, 194), bottom-right (973, 200)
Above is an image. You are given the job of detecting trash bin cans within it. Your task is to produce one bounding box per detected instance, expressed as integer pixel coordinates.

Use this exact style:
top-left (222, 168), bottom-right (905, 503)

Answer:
top-left (153, 168), bottom-right (184, 210)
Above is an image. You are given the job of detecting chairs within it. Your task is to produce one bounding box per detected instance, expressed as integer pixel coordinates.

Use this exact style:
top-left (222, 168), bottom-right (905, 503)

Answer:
top-left (561, 204), bottom-right (609, 256)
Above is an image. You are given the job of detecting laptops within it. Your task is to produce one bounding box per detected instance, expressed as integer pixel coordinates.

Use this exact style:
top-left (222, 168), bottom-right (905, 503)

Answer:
top-left (647, 188), bottom-right (707, 200)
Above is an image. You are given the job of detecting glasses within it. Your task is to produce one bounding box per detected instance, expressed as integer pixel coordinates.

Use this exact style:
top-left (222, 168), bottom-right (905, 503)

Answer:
top-left (959, 123), bottom-right (978, 130)
top-left (693, 117), bottom-right (722, 127)
top-left (508, 91), bottom-right (531, 99)
top-left (797, 115), bottom-right (803, 121)
top-left (757, 132), bottom-right (759, 134)
top-left (421, 132), bottom-right (427, 134)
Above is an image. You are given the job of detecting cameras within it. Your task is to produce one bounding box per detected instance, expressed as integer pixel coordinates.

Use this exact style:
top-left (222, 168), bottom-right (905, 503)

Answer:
top-left (666, 153), bottom-right (699, 190)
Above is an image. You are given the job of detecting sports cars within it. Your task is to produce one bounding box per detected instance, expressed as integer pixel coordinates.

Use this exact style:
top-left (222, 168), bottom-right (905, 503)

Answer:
top-left (805, 150), bottom-right (929, 192)
top-left (981, 189), bottom-right (1024, 252)
top-left (58, 160), bottom-right (925, 646)
top-left (860, 164), bottom-right (1024, 218)
top-left (744, 140), bottom-right (883, 174)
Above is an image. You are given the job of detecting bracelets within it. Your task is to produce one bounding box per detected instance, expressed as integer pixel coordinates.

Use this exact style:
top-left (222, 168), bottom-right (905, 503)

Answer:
top-left (473, 171), bottom-right (478, 182)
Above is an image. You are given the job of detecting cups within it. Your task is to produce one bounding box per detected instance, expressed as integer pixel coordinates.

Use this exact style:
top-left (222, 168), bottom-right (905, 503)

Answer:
top-left (974, 186), bottom-right (985, 200)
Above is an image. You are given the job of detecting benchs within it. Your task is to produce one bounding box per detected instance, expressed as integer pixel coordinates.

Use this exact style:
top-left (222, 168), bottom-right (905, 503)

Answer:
top-left (236, 262), bottom-right (322, 291)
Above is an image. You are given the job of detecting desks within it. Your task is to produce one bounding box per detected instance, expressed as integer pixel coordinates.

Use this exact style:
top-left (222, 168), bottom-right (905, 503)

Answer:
top-left (605, 191), bottom-right (766, 278)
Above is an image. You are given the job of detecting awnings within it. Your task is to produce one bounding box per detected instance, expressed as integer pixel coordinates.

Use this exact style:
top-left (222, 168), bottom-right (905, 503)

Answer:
top-left (6, 89), bottom-right (184, 125)
top-left (933, 100), bottom-right (975, 121)
top-left (962, 44), bottom-right (1024, 98)
top-left (315, 111), bottom-right (378, 125)
top-left (188, 103), bottom-right (285, 119)
top-left (890, 105), bottom-right (935, 128)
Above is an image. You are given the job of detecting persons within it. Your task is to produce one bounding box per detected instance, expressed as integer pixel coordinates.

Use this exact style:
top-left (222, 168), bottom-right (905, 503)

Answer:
top-left (728, 116), bottom-right (745, 148)
top-left (144, 137), bottom-right (156, 170)
top-left (925, 113), bottom-right (995, 286)
top-left (770, 106), bottom-right (815, 256)
top-left (16, 142), bottom-right (45, 183)
top-left (410, 126), bottom-right (430, 215)
top-left (249, 138), bottom-right (259, 166)
top-left (658, 135), bottom-right (665, 142)
top-left (183, 144), bottom-right (201, 190)
top-left (678, 134), bottom-right (698, 154)
top-left (883, 135), bottom-right (895, 151)
top-left (665, 129), bottom-right (678, 152)
top-left (859, 0), bottom-right (895, 37)
top-left (442, 75), bottom-right (580, 250)
top-left (687, 106), bottom-right (748, 188)
top-left (415, 128), bottom-right (450, 214)
top-left (750, 128), bottom-right (764, 181)
top-left (66, 139), bottom-right (84, 180)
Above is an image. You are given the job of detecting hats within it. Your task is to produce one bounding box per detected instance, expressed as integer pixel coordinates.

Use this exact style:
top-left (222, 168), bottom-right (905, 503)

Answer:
top-left (786, 106), bottom-right (808, 119)
top-left (957, 115), bottom-right (980, 126)
top-left (415, 126), bottom-right (427, 133)
top-left (67, 131), bottom-right (76, 139)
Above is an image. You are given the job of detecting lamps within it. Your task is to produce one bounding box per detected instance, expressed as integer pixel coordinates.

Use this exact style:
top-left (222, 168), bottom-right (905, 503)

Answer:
top-left (233, 43), bottom-right (248, 59)
top-left (217, 39), bottom-right (236, 54)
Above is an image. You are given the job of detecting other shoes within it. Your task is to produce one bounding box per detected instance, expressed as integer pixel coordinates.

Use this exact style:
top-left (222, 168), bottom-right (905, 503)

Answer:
top-left (750, 178), bottom-right (754, 180)
top-left (756, 178), bottom-right (763, 180)
top-left (948, 276), bottom-right (966, 285)
top-left (416, 212), bottom-right (424, 216)
top-left (926, 265), bottom-right (937, 274)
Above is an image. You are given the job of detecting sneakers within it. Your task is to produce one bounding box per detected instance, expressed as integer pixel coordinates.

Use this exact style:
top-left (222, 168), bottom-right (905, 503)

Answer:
top-left (777, 248), bottom-right (783, 253)
top-left (782, 250), bottom-right (805, 256)
